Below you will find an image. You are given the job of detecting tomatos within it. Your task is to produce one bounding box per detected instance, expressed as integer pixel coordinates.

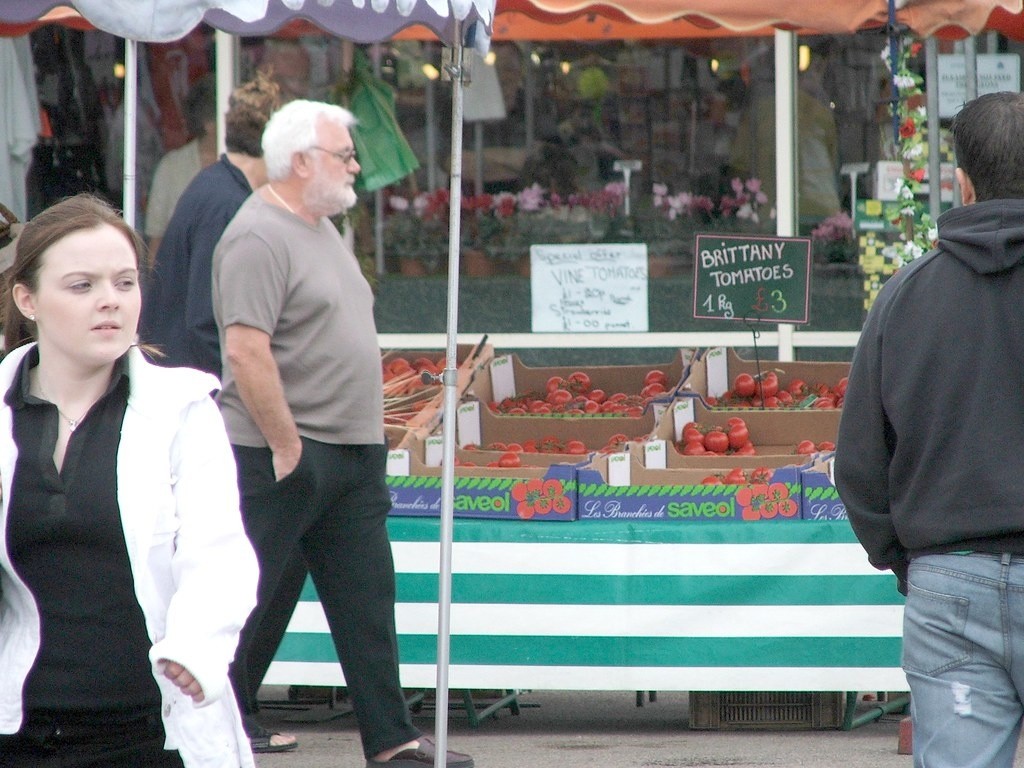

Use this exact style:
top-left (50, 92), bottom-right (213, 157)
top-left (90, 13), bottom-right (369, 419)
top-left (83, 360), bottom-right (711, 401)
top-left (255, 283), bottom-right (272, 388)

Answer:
top-left (669, 415), bottom-right (836, 484)
top-left (453, 433), bottom-right (651, 468)
top-left (486, 369), bottom-right (667, 414)
top-left (704, 370), bottom-right (852, 409)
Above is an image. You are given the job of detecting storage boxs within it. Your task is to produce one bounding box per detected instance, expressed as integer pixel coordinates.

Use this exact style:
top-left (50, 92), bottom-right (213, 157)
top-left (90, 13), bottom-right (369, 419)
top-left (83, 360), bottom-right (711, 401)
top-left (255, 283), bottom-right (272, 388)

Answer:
top-left (690, 690), bottom-right (845, 732)
top-left (376, 344), bottom-right (851, 522)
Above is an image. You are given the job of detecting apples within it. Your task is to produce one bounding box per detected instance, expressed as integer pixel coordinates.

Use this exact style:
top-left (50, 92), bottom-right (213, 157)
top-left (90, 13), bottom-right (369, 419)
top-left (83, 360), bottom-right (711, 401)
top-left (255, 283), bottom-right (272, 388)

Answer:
top-left (381, 356), bottom-right (464, 428)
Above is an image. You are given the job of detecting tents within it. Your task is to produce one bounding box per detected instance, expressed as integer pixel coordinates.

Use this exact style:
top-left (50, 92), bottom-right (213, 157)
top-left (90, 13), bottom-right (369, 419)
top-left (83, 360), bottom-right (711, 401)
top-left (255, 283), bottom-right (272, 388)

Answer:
top-left (0, 2), bottom-right (1024, 768)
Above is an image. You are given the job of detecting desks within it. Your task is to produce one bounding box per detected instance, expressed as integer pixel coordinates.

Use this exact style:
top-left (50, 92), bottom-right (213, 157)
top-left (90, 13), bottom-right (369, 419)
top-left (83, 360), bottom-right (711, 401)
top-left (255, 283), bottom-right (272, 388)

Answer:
top-left (256, 519), bottom-right (909, 737)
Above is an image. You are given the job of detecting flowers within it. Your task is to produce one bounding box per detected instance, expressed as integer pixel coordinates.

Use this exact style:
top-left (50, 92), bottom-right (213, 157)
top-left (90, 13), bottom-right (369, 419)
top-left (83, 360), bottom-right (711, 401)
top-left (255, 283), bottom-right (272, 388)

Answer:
top-left (881, 33), bottom-right (939, 274)
top-left (359, 172), bottom-right (859, 276)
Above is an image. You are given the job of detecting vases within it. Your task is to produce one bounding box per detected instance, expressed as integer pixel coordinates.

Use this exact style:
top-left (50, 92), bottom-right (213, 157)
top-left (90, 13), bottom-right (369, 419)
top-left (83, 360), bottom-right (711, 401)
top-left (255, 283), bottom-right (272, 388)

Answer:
top-left (398, 255), bottom-right (425, 277)
top-left (462, 249), bottom-right (497, 276)
top-left (649, 254), bottom-right (674, 278)
top-left (511, 252), bottom-right (531, 276)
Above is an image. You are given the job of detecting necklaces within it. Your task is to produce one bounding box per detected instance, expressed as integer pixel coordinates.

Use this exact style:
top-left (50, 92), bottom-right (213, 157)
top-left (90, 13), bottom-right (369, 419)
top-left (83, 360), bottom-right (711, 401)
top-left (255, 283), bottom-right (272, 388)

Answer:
top-left (36, 376), bottom-right (99, 432)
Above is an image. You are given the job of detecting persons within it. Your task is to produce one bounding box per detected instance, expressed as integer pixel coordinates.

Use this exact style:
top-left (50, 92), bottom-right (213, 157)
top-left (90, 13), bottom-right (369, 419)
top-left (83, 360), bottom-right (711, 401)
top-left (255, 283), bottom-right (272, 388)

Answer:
top-left (735, 78), bottom-right (840, 238)
top-left (0, 195), bottom-right (261, 768)
top-left (137, 79), bottom-right (302, 397)
top-left (832, 90), bottom-right (1024, 768)
top-left (212, 98), bottom-right (476, 768)
top-left (145, 73), bottom-right (217, 268)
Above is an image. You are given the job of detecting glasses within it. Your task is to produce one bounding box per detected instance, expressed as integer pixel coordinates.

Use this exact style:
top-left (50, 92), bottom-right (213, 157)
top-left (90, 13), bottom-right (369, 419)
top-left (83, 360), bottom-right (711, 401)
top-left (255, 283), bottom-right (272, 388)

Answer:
top-left (308, 145), bottom-right (357, 165)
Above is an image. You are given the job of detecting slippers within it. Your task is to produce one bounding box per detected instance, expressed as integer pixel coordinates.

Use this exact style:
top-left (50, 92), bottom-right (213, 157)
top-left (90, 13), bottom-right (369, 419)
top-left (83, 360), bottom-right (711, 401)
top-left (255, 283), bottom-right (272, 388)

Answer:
top-left (365, 733), bottom-right (474, 768)
top-left (239, 715), bottom-right (299, 753)
